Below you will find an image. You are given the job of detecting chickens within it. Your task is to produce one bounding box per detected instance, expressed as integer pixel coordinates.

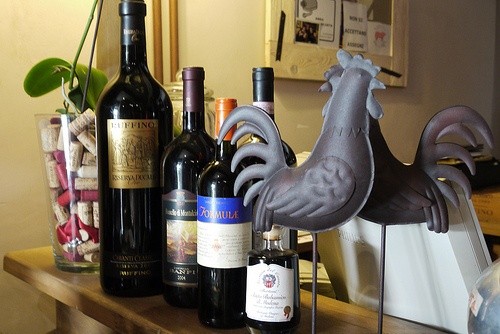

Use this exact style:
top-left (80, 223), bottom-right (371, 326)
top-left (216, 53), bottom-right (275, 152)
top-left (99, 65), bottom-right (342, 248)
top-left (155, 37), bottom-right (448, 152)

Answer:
top-left (214, 47), bottom-right (497, 235)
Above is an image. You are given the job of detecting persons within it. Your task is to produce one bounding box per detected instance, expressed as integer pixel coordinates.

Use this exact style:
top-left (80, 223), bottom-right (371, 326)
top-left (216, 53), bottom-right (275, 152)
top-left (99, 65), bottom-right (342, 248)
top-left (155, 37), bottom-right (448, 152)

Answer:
top-left (296, 24), bottom-right (317, 44)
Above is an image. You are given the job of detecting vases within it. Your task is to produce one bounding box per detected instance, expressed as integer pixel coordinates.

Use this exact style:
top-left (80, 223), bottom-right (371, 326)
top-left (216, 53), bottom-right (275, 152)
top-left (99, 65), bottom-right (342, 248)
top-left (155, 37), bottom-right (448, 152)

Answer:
top-left (34, 112), bottom-right (101, 274)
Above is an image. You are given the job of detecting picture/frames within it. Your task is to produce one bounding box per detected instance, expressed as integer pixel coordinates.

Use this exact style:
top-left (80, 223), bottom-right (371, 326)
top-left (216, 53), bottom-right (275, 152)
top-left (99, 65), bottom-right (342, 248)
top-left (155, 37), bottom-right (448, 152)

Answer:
top-left (264, 0), bottom-right (409, 86)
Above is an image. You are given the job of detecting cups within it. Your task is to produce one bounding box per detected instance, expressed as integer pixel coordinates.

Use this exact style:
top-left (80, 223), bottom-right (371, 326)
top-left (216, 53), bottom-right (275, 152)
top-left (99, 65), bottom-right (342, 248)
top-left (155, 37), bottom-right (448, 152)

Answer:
top-left (34, 112), bottom-right (100, 273)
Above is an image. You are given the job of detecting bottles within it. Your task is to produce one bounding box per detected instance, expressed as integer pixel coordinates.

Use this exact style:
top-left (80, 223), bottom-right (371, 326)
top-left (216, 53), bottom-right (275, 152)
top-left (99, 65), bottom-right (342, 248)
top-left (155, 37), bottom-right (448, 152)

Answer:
top-left (195, 98), bottom-right (253, 330)
top-left (95, 0), bottom-right (173, 297)
top-left (244, 225), bottom-right (301, 334)
top-left (238, 67), bottom-right (298, 252)
top-left (161, 66), bottom-right (215, 309)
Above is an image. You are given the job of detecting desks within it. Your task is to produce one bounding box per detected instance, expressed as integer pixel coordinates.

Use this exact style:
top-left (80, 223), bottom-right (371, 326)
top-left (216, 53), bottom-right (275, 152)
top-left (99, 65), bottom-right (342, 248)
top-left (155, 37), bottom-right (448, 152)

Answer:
top-left (3, 246), bottom-right (449, 334)
